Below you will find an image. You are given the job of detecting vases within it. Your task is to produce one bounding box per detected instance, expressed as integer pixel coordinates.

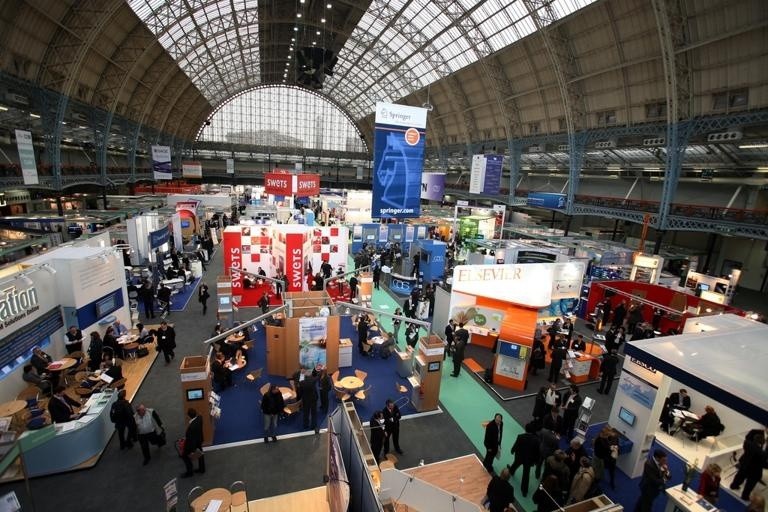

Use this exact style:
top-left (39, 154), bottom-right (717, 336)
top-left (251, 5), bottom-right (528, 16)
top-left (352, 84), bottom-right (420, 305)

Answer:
top-left (681, 470), bottom-right (692, 491)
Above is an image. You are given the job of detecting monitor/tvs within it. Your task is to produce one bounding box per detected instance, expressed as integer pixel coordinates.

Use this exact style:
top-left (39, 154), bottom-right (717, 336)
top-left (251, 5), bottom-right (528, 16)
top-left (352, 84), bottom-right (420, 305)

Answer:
top-left (619, 406), bottom-right (636, 426)
top-left (428, 361), bottom-right (440, 371)
top-left (700, 283), bottom-right (710, 291)
top-left (714, 283), bottom-right (727, 294)
top-left (181, 221), bottom-right (189, 228)
top-left (420, 248), bottom-right (430, 264)
top-left (187, 388), bottom-right (204, 400)
top-left (96, 292), bottom-right (118, 321)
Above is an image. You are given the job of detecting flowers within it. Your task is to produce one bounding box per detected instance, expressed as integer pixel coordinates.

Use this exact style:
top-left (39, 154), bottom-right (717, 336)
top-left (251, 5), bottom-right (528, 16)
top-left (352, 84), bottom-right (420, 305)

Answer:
top-left (682, 457), bottom-right (699, 472)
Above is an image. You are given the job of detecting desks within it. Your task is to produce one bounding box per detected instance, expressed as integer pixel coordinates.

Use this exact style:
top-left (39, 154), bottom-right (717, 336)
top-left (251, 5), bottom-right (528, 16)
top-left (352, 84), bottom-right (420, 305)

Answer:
top-left (0, 399), bottom-right (28, 419)
top-left (663, 482), bottom-right (720, 512)
top-left (671, 408), bottom-right (699, 436)
top-left (115, 334), bottom-right (139, 345)
top-left (45, 358), bottom-right (78, 385)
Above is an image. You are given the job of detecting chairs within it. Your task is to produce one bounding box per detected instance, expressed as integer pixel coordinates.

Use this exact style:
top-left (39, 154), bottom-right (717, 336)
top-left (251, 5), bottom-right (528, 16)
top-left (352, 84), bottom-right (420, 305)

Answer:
top-left (351, 312), bottom-right (396, 358)
top-left (188, 480), bottom-right (249, 511)
top-left (330, 369), bottom-right (373, 405)
top-left (65, 343), bottom-right (139, 394)
top-left (259, 379), bottom-right (303, 418)
top-left (680, 422), bottom-right (725, 450)
top-left (245, 367), bottom-right (263, 385)
top-left (15, 385), bottom-right (51, 429)
top-left (395, 382), bottom-right (408, 397)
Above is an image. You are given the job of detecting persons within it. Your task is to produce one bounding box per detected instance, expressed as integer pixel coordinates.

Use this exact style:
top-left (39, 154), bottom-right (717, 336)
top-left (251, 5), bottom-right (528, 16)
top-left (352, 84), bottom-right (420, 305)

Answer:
top-left (1, 186), bottom-right (767, 509)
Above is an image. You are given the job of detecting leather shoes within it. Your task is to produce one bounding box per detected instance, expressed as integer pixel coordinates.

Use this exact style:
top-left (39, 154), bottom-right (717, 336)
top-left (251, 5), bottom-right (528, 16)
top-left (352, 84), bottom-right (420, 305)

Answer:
top-left (264, 437), bottom-right (268, 443)
top-left (142, 455), bottom-right (151, 465)
top-left (194, 469), bottom-right (205, 473)
top-left (272, 435), bottom-right (277, 442)
top-left (179, 472), bottom-right (193, 478)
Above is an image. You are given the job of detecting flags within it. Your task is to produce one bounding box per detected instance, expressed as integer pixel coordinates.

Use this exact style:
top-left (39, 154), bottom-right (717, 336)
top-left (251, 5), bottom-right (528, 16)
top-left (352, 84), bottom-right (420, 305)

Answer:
top-left (371, 101), bottom-right (429, 218)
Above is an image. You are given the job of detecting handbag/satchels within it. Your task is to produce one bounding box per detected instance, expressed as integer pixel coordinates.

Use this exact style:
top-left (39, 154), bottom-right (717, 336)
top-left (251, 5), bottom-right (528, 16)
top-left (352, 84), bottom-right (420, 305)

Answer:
top-left (155, 426), bottom-right (166, 448)
top-left (137, 347), bottom-right (149, 358)
top-left (583, 478), bottom-right (604, 500)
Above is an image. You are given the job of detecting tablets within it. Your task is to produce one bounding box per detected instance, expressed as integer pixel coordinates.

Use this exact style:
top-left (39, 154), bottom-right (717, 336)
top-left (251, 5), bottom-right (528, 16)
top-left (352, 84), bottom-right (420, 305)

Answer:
top-left (694, 498), bottom-right (718, 512)
